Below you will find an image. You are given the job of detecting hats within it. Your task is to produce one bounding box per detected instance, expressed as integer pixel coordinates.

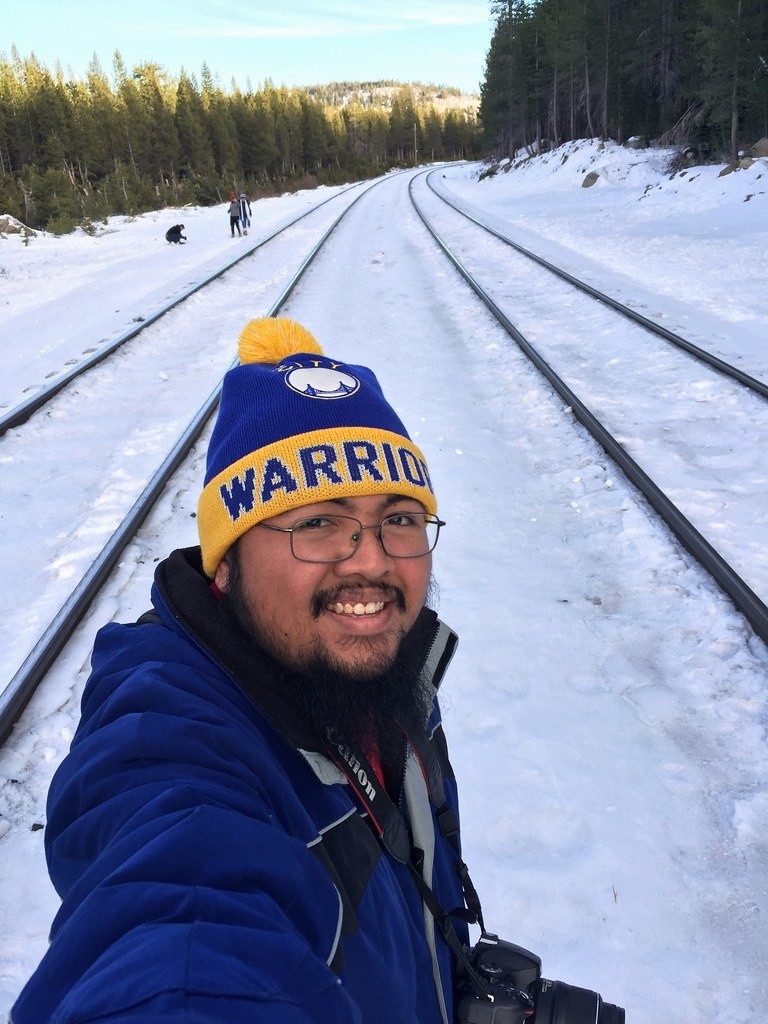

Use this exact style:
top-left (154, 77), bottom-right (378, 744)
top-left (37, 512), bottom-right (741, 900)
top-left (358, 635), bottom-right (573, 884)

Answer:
top-left (197, 317), bottom-right (438, 579)
top-left (239, 193), bottom-right (247, 197)
top-left (230, 194), bottom-right (236, 199)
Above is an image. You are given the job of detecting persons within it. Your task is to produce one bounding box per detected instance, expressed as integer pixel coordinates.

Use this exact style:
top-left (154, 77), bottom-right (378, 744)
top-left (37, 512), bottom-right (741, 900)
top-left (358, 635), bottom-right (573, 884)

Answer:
top-left (11, 319), bottom-right (472, 1024)
top-left (239, 194), bottom-right (252, 235)
top-left (166, 224), bottom-right (187, 245)
top-left (228, 193), bottom-right (242, 237)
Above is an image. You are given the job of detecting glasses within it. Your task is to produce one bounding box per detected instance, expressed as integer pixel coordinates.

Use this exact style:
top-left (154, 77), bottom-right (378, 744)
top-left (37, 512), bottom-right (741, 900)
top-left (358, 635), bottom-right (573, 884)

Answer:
top-left (256, 512), bottom-right (447, 564)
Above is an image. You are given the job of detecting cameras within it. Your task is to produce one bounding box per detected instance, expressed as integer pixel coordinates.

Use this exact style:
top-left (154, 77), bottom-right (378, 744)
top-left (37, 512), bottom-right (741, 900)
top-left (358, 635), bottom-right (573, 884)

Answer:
top-left (453, 933), bottom-right (626, 1024)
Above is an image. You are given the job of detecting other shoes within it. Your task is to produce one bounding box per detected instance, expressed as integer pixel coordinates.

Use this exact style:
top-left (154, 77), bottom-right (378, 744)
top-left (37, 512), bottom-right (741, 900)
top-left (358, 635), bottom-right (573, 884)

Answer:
top-left (179, 242), bottom-right (185, 244)
top-left (238, 234), bottom-right (243, 238)
top-left (243, 229), bottom-right (248, 235)
top-left (231, 234), bottom-right (236, 238)
